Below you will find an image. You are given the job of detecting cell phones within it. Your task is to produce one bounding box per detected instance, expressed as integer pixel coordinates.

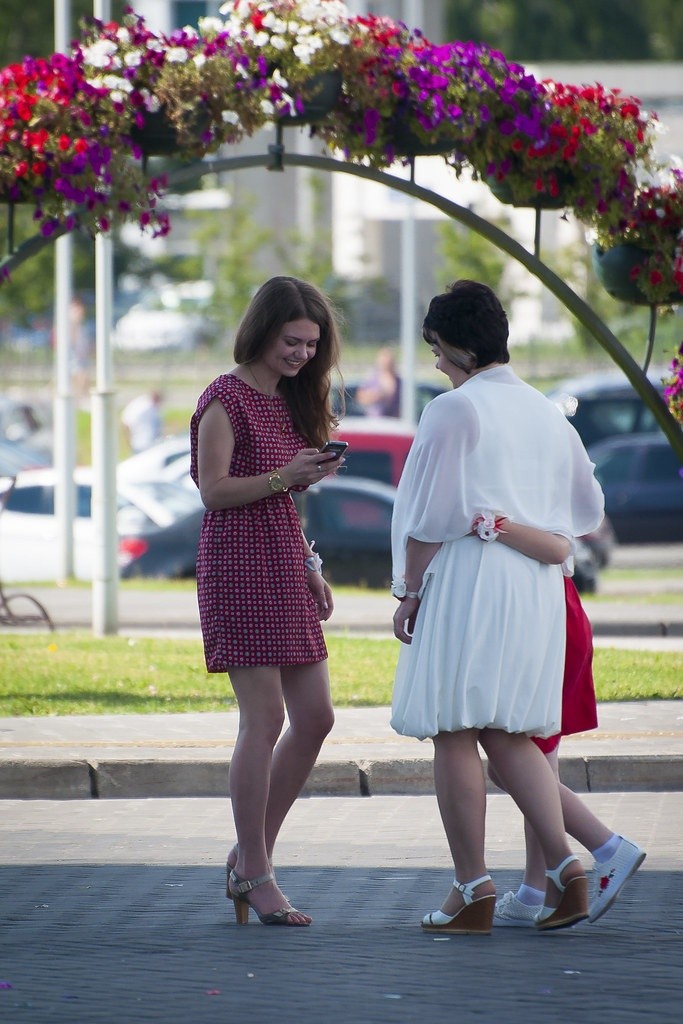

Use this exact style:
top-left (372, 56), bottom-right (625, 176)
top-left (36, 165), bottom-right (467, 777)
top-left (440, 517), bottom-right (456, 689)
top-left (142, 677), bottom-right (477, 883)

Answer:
top-left (316, 441), bottom-right (348, 464)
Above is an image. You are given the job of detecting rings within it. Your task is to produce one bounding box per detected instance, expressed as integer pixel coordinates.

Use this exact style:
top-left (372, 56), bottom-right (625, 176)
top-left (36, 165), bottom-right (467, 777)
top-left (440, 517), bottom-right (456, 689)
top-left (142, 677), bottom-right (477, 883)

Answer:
top-left (318, 463), bottom-right (321, 472)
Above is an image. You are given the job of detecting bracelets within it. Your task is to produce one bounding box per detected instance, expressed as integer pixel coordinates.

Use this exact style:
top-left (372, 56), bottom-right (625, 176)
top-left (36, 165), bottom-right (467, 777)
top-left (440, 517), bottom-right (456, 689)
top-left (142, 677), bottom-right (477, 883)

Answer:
top-left (472, 511), bottom-right (508, 544)
top-left (305, 540), bottom-right (323, 571)
top-left (391, 575), bottom-right (418, 602)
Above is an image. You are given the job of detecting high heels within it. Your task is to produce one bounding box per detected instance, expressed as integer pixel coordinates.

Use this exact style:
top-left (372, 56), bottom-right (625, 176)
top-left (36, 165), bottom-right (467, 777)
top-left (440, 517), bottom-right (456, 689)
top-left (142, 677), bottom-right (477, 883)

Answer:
top-left (421, 874), bottom-right (496, 934)
top-left (226, 844), bottom-right (290, 903)
top-left (533, 855), bottom-right (589, 930)
top-left (230, 870), bottom-right (311, 926)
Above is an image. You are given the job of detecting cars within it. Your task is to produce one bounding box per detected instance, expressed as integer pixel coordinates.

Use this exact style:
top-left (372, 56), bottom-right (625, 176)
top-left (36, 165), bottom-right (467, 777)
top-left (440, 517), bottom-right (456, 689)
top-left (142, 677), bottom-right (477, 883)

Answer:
top-left (547, 370), bottom-right (683, 546)
top-left (0, 383), bottom-right (613, 595)
top-left (114, 281), bottom-right (260, 354)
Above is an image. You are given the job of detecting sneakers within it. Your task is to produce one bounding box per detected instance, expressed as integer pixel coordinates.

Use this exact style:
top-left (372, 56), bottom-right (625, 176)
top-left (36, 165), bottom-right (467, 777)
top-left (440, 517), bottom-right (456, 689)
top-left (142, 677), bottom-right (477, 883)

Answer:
top-left (493, 892), bottom-right (544, 926)
top-left (587, 835), bottom-right (648, 922)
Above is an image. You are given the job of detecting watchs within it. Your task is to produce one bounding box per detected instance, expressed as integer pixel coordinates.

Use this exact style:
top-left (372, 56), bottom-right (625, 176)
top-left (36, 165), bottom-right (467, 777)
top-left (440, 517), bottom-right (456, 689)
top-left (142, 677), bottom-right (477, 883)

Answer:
top-left (269, 470), bottom-right (285, 493)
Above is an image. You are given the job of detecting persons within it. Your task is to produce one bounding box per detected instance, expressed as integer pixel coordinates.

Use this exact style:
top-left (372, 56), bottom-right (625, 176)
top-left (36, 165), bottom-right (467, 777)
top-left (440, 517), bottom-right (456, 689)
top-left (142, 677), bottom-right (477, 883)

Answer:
top-left (466, 515), bottom-right (647, 924)
top-left (191, 276), bottom-right (346, 927)
top-left (390, 277), bottom-right (605, 936)
top-left (123, 386), bottom-right (166, 453)
top-left (357, 347), bottom-right (401, 416)
